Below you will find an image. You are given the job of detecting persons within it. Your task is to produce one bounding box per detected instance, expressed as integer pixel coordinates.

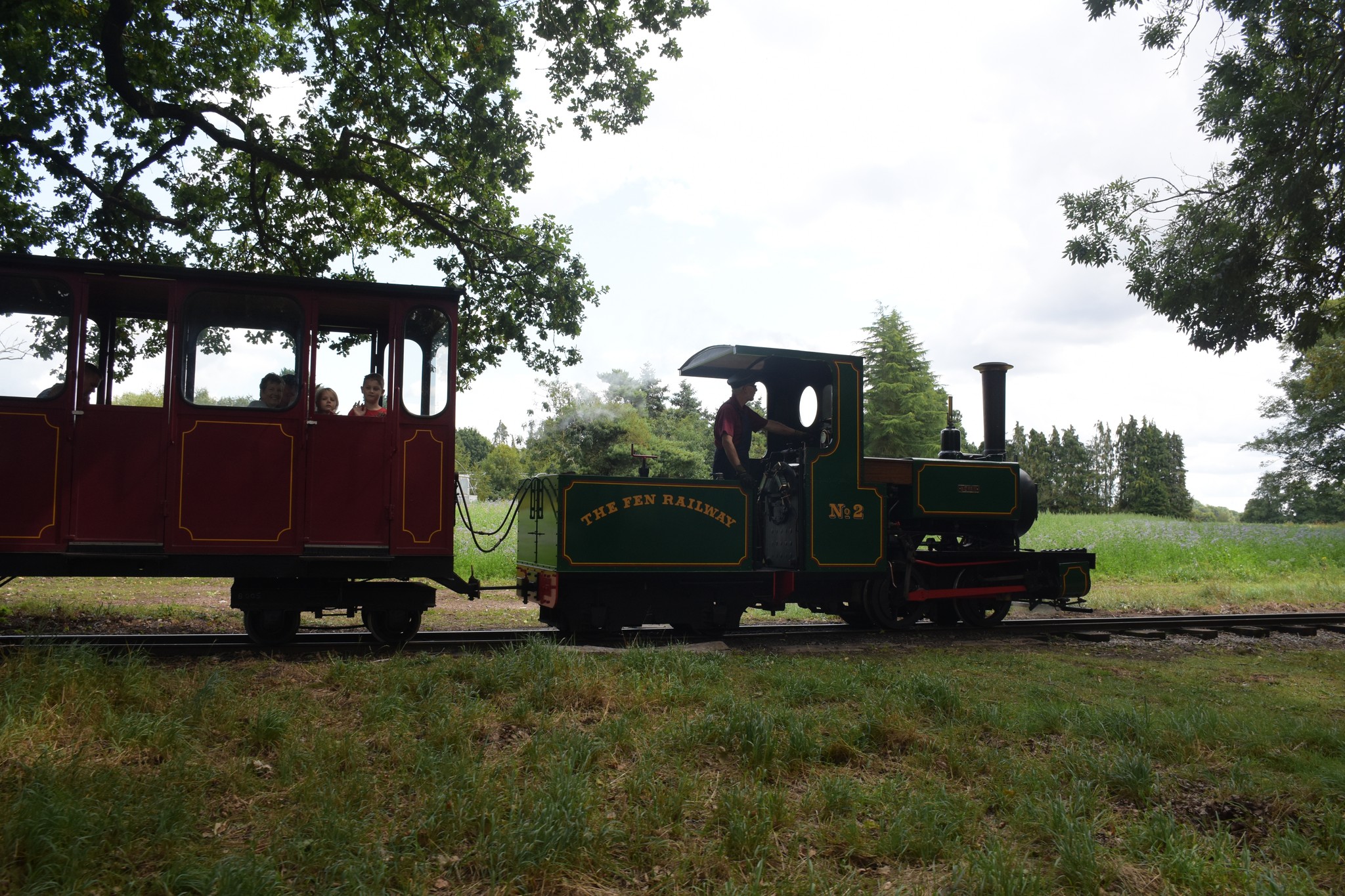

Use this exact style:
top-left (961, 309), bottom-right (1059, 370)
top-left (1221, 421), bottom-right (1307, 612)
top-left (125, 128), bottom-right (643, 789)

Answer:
top-left (36, 361), bottom-right (104, 401)
top-left (712, 371), bottom-right (807, 490)
top-left (315, 387), bottom-right (339, 415)
top-left (277, 374), bottom-right (298, 409)
top-left (248, 372), bottom-right (286, 408)
top-left (348, 373), bottom-right (387, 417)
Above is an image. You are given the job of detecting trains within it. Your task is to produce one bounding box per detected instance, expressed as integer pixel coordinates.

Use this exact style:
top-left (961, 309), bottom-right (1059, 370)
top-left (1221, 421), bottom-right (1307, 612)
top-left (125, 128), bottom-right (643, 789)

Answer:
top-left (0, 251), bottom-right (1097, 664)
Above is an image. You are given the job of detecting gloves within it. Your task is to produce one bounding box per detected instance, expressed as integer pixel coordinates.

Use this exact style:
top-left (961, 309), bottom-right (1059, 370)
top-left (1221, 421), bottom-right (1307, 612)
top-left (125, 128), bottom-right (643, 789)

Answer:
top-left (736, 465), bottom-right (753, 486)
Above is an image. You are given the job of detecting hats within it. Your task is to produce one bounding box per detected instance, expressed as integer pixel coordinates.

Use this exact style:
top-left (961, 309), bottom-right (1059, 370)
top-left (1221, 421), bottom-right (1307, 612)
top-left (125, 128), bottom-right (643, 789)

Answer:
top-left (727, 370), bottom-right (759, 388)
